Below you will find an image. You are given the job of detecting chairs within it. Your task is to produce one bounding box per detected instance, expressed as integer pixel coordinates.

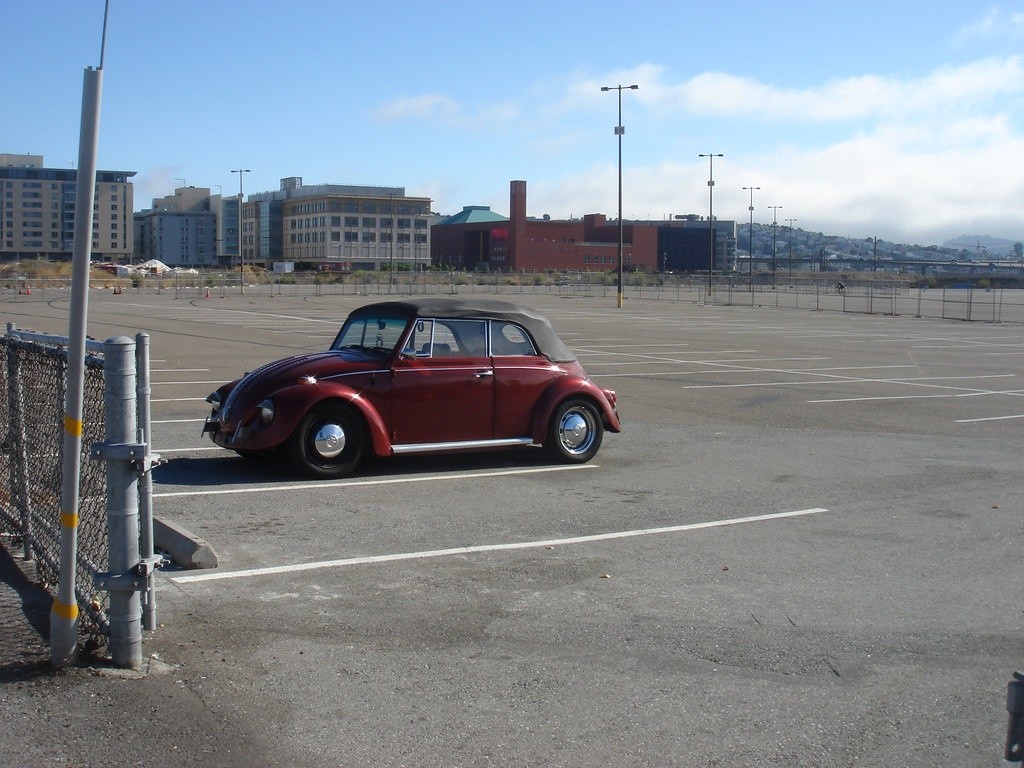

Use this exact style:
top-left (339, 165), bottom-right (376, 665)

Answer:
top-left (422, 342), bottom-right (451, 357)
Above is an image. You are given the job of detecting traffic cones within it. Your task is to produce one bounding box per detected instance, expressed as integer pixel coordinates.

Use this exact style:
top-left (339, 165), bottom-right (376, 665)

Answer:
top-left (26, 286), bottom-right (31, 295)
top-left (113, 285), bottom-right (123, 294)
top-left (205, 288), bottom-right (210, 298)
top-left (19, 287), bottom-right (23, 296)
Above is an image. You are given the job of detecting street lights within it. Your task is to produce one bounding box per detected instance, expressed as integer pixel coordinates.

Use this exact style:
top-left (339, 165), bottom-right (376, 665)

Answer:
top-left (785, 218), bottom-right (799, 291)
top-left (232, 168), bottom-right (252, 297)
top-left (699, 153), bottom-right (723, 299)
top-left (601, 85), bottom-right (638, 308)
top-left (769, 205), bottom-right (782, 288)
top-left (743, 186), bottom-right (761, 290)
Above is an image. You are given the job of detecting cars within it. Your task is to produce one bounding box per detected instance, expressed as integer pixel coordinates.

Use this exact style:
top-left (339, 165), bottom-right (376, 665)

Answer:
top-left (199, 298), bottom-right (621, 479)
